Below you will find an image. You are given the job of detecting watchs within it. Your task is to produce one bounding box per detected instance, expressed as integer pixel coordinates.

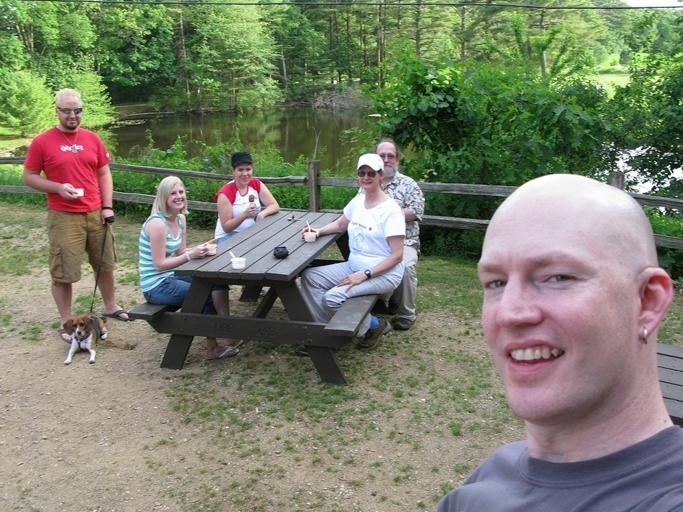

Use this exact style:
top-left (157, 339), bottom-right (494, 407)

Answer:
top-left (364, 268), bottom-right (371, 278)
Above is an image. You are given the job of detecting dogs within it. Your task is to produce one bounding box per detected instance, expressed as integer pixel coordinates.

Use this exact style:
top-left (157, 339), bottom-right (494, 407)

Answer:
top-left (62, 314), bottom-right (109, 365)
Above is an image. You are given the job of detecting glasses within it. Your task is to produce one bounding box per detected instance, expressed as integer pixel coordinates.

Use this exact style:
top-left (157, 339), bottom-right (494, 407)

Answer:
top-left (380, 153), bottom-right (395, 159)
top-left (57, 106), bottom-right (83, 114)
top-left (358, 172), bottom-right (377, 177)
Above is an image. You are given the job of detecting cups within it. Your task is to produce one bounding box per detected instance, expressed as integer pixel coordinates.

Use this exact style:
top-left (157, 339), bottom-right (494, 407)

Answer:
top-left (71, 188), bottom-right (83, 197)
top-left (273, 247), bottom-right (286, 260)
top-left (207, 245), bottom-right (217, 255)
top-left (303, 232), bottom-right (316, 243)
top-left (231, 258), bottom-right (245, 268)
top-left (249, 204), bottom-right (257, 218)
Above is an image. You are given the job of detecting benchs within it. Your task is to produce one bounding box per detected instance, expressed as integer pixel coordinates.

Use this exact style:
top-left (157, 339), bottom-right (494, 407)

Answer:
top-left (127, 302), bottom-right (184, 323)
top-left (322, 293), bottom-right (378, 337)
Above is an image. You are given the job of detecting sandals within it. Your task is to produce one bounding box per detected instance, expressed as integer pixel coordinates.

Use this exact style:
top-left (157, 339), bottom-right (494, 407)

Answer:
top-left (102, 306), bottom-right (136, 322)
top-left (58, 318), bottom-right (76, 344)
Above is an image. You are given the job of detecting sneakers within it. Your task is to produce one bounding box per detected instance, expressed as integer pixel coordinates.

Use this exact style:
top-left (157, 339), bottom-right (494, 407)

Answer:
top-left (394, 309), bottom-right (416, 331)
top-left (359, 315), bottom-right (392, 351)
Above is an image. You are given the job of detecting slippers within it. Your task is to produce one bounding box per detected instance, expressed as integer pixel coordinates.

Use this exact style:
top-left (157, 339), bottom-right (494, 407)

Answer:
top-left (206, 345), bottom-right (240, 360)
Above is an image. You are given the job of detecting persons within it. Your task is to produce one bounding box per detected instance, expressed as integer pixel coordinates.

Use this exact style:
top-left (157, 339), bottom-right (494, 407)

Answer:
top-left (215, 151), bottom-right (279, 243)
top-left (358, 135), bottom-right (425, 331)
top-left (140, 174), bottom-right (249, 360)
top-left (430, 171), bottom-right (683, 512)
top-left (21, 88), bottom-right (134, 344)
top-left (301, 153), bottom-right (406, 352)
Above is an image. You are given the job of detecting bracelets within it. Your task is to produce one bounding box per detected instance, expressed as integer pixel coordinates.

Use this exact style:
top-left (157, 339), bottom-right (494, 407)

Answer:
top-left (185, 249), bottom-right (191, 262)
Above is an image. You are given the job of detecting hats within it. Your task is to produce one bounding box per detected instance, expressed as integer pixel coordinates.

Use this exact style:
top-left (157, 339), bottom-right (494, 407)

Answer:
top-left (356, 153), bottom-right (385, 171)
top-left (231, 152), bottom-right (254, 169)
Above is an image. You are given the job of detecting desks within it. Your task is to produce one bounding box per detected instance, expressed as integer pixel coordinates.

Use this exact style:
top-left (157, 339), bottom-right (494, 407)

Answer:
top-left (159, 210), bottom-right (350, 384)
